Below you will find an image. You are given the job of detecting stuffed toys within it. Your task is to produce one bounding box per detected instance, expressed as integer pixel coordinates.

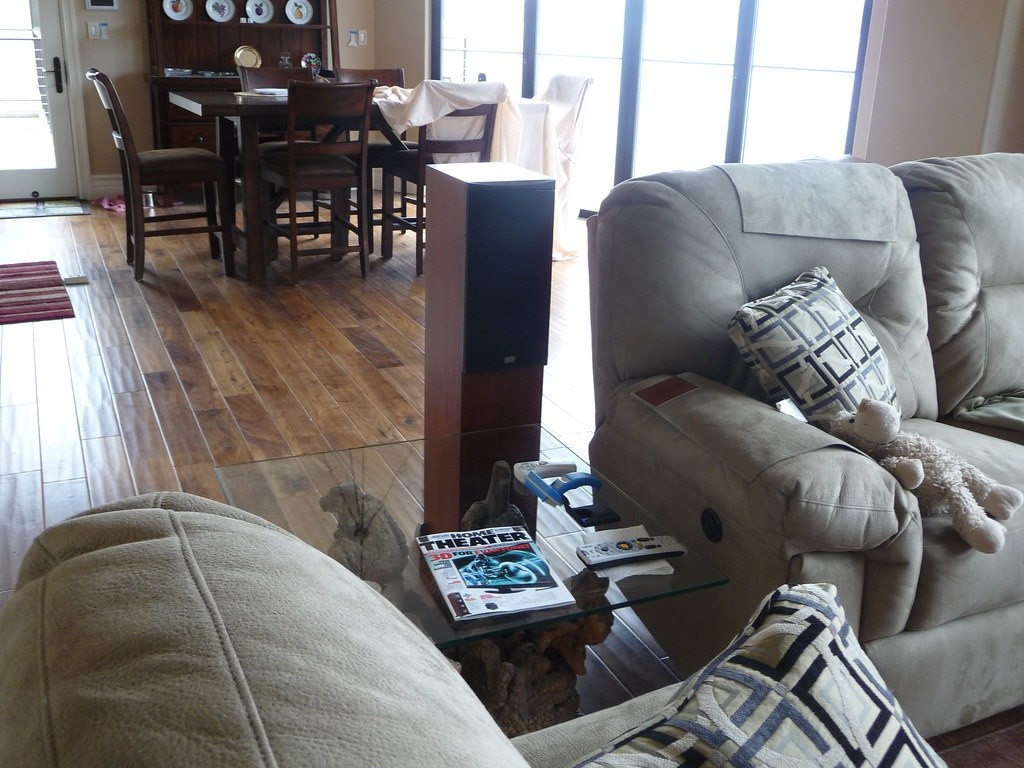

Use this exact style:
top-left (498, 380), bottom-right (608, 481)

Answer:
top-left (832, 399), bottom-right (1023, 554)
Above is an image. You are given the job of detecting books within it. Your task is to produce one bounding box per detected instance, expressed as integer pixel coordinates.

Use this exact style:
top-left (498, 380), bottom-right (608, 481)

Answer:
top-left (414, 526), bottom-right (575, 621)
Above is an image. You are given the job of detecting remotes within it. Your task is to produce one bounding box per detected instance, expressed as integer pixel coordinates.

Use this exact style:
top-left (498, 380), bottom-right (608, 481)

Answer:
top-left (512, 460), bottom-right (577, 482)
top-left (576, 536), bottom-right (687, 571)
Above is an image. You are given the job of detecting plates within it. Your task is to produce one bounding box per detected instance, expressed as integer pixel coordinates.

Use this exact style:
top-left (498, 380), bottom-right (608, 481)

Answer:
top-left (254, 88), bottom-right (286, 95)
top-left (162, 0), bottom-right (194, 21)
top-left (285, 0), bottom-right (314, 24)
top-left (164, 68), bottom-right (192, 76)
top-left (234, 45), bottom-right (261, 68)
top-left (246, 0), bottom-right (274, 23)
top-left (301, 54), bottom-right (321, 75)
top-left (205, 0), bottom-right (235, 23)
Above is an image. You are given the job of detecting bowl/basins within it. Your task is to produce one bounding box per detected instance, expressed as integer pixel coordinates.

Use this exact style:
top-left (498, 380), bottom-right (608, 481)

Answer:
top-left (140, 190), bottom-right (162, 207)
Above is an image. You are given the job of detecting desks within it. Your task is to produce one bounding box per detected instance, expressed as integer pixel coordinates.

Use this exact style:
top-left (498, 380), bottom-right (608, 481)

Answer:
top-left (215, 423), bottom-right (731, 742)
top-left (169, 90), bottom-right (395, 282)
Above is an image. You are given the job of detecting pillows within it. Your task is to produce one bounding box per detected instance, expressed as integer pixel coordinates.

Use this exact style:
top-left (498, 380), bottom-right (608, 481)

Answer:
top-left (728, 267), bottom-right (904, 424)
top-left (565, 583), bottom-right (948, 768)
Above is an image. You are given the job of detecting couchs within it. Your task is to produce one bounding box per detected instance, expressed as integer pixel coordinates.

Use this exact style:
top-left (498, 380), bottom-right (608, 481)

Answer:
top-left (585, 152), bottom-right (1024, 740)
top-left (0, 492), bottom-right (689, 768)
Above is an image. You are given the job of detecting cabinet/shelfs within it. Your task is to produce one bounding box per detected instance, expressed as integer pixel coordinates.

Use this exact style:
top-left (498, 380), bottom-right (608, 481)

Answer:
top-left (144, 0), bottom-right (350, 207)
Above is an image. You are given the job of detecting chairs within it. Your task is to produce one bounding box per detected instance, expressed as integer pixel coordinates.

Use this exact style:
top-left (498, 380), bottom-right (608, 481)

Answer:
top-left (85, 67), bottom-right (235, 280)
top-left (333, 68), bottom-right (405, 253)
top-left (382, 80), bottom-right (505, 275)
top-left (258, 78), bottom-right (378, 284)
top-left (238, 67), bottom-right (320, 238)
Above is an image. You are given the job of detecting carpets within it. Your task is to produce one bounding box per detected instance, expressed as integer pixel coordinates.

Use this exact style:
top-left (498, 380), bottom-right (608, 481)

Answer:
top-left (0, 261), bottom-right (75, 325)
top-left (0, 198), bottom-right (91, 219)
